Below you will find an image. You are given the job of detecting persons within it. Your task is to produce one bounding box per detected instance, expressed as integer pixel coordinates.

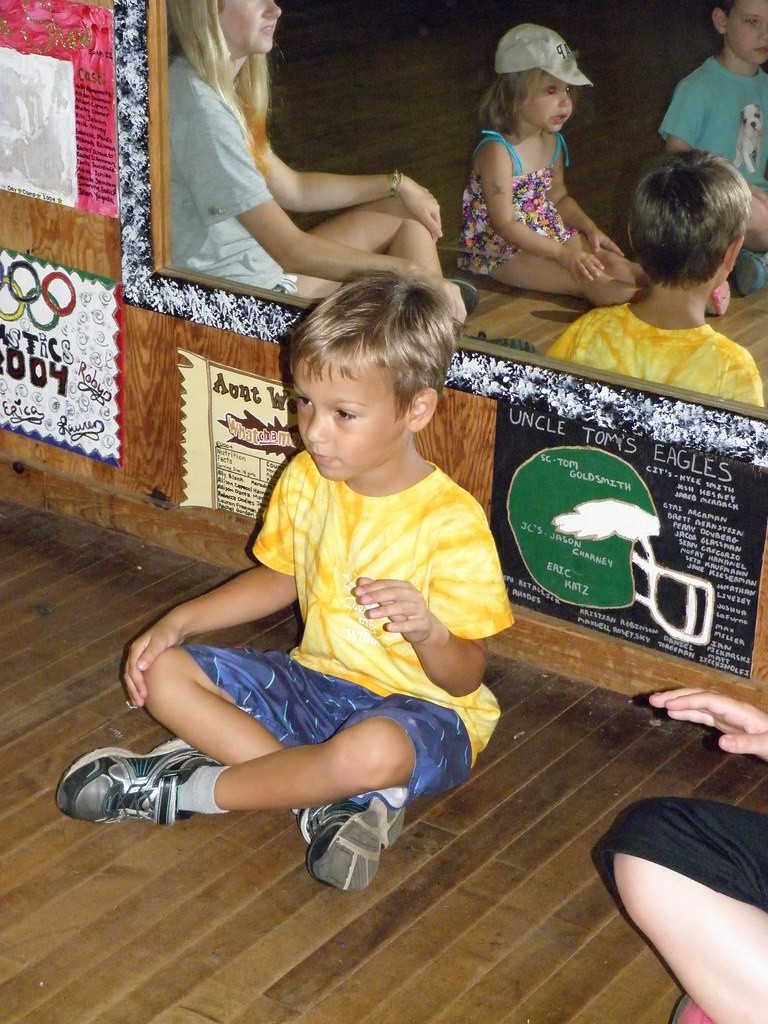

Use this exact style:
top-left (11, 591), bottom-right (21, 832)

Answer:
top-left (599, 688), bottom-right (768, 1024)
top-left (457, 24), bottom-right (729, 315)
top-left (54, 268), bottom-right (517, 892)
top-left (546, 148), bottom-right (764, 408)
top-left (662, 0), bottom-right (768, 296)
top-left (167, 0), bottom-right (535, 353)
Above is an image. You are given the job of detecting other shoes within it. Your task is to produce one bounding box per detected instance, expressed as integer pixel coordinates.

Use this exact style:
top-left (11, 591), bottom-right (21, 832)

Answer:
top-left (446, 279), bottom-right (480, 316)
top-left (705, 280), bottom-right (730, 315)
top-left (464, 331), bottom-right (536, 353)
top-left (731, 248), bottom-right (768, 296)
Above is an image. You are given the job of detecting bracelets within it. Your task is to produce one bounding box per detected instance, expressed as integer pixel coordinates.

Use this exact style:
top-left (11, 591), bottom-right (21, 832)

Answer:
top-left (390, 170), bottom-right (403, 197)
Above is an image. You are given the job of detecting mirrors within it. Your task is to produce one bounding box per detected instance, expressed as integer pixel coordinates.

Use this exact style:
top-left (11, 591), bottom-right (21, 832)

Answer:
top-left (110, 0), bottom-right (768, 463)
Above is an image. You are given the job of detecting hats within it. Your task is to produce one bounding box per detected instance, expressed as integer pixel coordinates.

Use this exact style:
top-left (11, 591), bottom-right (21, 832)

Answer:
top-left (494, 24), bottom-right (594, 88)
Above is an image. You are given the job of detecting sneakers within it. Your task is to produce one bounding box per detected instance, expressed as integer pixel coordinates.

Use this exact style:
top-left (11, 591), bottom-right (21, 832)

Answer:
top-left (296, 786), bottom-right (409, 892)
top-left (56, 737), bottom-right (222, 826)
